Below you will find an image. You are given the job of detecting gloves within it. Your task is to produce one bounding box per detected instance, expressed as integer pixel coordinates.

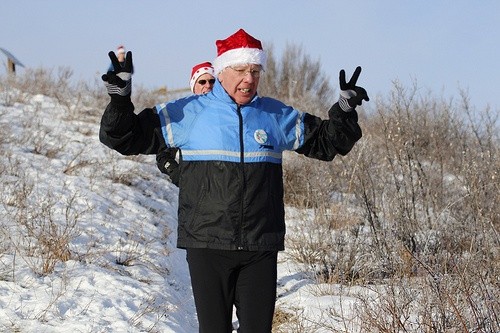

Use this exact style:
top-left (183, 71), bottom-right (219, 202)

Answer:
top-left (336, 65), bottom-right (369, 116)
top-left (165, 161), bottom-right (180, 187)
top-left (103, 50), bottom-right (133, 106)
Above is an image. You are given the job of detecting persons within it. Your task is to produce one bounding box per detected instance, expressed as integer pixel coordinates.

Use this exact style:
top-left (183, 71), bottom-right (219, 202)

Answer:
top-left (157, 61), bottom-right (215, 189)
top-left (107, 45), bottom-right (126, 74)
top-left (98, 27), bottom-right (370, 333)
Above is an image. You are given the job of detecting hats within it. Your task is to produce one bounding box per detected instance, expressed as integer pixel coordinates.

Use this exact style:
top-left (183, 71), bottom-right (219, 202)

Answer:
top-left (190, 62), bottom-right (216, 94)
top-left (118, 46), bottom-right (124, 53)
top-left (214, 28), bottom-right (265, 74)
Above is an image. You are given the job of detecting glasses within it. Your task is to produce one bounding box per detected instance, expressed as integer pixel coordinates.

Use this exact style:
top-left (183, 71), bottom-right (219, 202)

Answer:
top-left (196, 79), bottom-right (216, 84)
top-left (230, 65), bottom-right (265, 77)
